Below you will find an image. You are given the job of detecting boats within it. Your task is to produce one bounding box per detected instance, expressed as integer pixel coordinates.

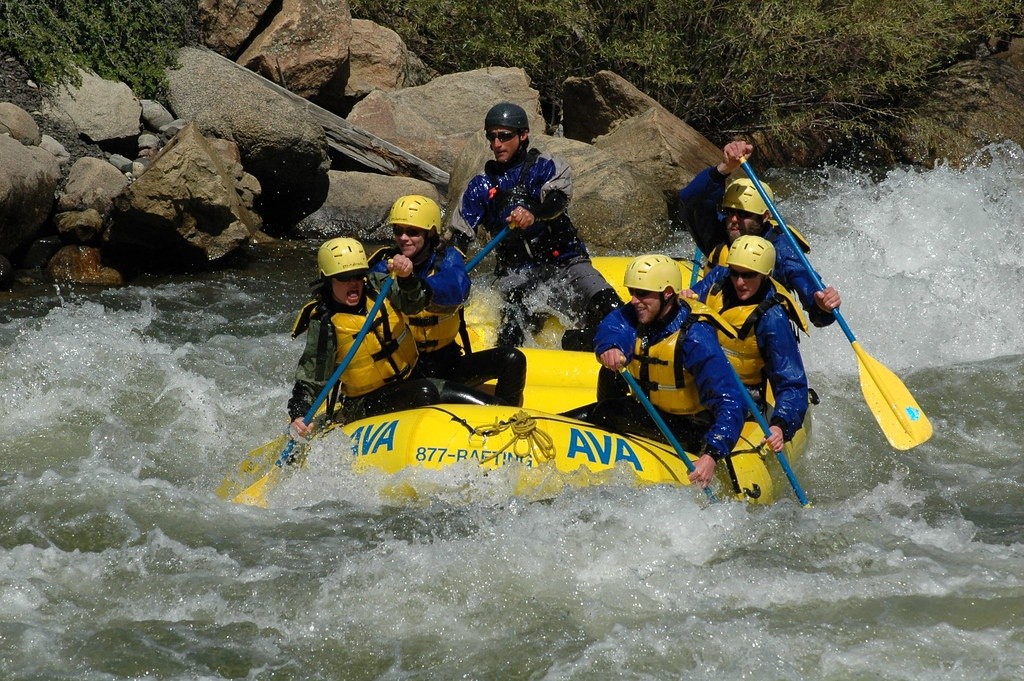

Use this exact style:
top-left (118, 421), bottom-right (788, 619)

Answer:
top-left (285, 254), bottom-right (813, 509)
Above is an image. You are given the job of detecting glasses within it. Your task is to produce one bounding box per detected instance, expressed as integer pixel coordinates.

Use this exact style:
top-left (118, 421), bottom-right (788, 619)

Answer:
top-left (728, 265), bottom-right (759, 280)
top-left (332, 270), bottom-right (366, 283)
top-left (628, 287), bottom-right (653, 299)
top-left (393, 227), bottom-right (425, 237)
top-left (719, 206), bottom-right (755, 219)
top-left (485, 130), bottom-right (521, 142)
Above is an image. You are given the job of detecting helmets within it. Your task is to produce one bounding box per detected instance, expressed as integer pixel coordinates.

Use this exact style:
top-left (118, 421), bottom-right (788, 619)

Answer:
top-left (726, 235), bottom-right (776, 277)
top-left (318, 236), bottom-right (369, 280)
top-left (389, 195), bottom-right (441, 235)
top-left (721, 177), bottom-right (775, 218)
top-left (624, 253), bottom-right (682, 296)
top-left (484, 103), bottom-right (530, 134)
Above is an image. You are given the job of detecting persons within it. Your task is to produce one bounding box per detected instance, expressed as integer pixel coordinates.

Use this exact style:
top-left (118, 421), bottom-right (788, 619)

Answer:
top-left (287, 238), bottom-right (441, 442)
top-left (675, 140), bottom-right (842, 327)
top-left (367, 195), bottom-right (527, 406)
top-left (556, 254), bottom-right (748, 488)
top-left (447, 103), bottom-right (625, 348)
top-left (680, 235), bottom-right (809, 452)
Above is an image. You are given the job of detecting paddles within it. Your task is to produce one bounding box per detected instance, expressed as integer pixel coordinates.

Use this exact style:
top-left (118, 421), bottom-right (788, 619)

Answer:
top-left (215, 421), bottom-right (291, 500)
top-left (231, 259), bottom-right (399, 506)
top-left (692, 292), bottom-right (811, 508)
top-left (740, 155), bottom-right (934, 450)
top-left (465, 211), bottom-right (516, 274)
top-left (617, 356), bottom-right (718, 503)
